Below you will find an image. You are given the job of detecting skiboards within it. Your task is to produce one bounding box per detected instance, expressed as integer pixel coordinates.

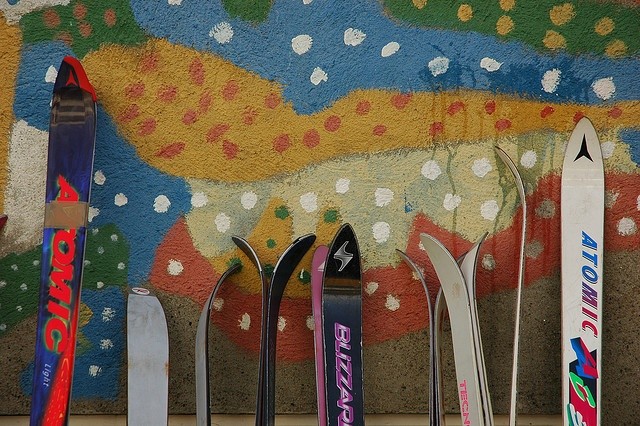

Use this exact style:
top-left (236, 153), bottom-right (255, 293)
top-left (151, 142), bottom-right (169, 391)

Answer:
top-left (232, 233), bottom-right (317, 426)
top-left (395, 249), bottom-right (469, 425)
top-left (560, 115), bottom-right (604, 426)
top-left (196, 263), bottom-right (240, 426)
top-left (420, 231), bottom-right (496, 426)
top-left (29, 56), bottom-right (97, 426)
top-left (322, 222), bottom-right (367, 426)
top-left (495, 146), bottom-right (528, 426)
top-left (127, 288), bottom-right (170, 426)
top-left (311, 244), bottom-right (328, 426)
